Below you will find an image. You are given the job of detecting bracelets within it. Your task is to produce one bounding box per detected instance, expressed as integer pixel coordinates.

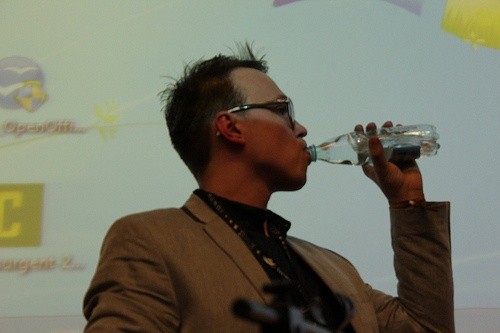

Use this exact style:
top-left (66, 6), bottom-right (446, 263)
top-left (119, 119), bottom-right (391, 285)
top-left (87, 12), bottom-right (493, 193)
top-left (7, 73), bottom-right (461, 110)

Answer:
top-left (388, 196), bottom-right (425, 208)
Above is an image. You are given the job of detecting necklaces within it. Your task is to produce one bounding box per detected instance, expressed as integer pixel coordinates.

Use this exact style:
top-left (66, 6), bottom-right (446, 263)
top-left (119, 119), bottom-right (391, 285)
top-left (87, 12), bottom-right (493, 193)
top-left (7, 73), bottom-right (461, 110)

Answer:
top-left (210, 191), bottom-right (304, 292)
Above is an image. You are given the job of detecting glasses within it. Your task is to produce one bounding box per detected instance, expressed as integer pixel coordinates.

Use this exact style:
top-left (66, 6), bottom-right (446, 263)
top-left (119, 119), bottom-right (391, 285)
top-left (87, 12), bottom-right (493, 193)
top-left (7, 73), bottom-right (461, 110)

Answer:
top-left (229, 98), bottom-right (298, 130)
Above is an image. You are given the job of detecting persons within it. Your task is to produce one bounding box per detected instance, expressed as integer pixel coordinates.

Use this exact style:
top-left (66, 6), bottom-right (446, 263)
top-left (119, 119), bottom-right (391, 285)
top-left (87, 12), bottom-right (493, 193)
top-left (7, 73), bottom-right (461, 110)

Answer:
top-left (82, 39), bottom-right (458, 333)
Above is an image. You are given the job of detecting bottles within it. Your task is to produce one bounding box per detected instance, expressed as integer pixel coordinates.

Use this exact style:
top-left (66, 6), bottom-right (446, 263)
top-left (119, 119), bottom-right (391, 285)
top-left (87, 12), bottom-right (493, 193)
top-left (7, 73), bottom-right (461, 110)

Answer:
top-left (304, 124), bottom-right (440, 167)
